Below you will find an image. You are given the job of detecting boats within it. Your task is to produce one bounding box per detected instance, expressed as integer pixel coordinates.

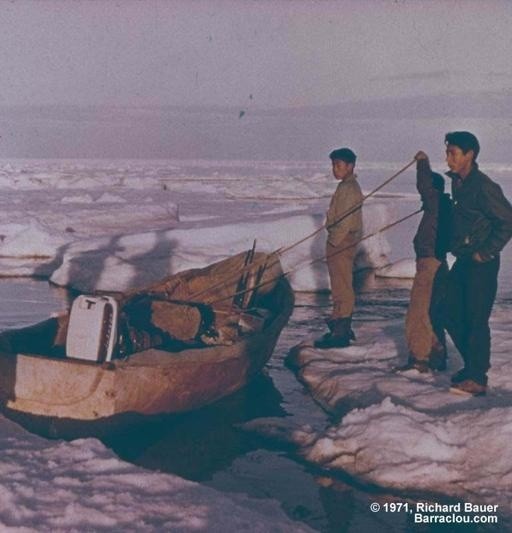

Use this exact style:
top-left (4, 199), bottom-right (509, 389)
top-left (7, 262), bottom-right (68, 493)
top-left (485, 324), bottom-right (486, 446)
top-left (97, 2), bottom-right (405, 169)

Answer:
top-left (0, 250), bottom-right (296, 438)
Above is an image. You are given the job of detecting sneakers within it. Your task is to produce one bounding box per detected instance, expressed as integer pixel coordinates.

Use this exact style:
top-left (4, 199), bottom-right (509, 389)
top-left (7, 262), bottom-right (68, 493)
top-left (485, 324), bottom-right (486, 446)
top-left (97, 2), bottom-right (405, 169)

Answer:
top-left (450, 368), bottom-right (467, 380)
top-left (448, 378), bottom-right (489, 396)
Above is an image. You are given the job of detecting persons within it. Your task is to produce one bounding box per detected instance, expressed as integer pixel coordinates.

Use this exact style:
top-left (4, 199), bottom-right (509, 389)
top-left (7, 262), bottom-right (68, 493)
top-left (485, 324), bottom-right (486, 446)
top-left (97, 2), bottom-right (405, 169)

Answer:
top-left (442, 132), bottom-right (512, 396)
top-left (396, 151), bottom-right (451, 374)
top-left (313, 148), bottom-right (362, 348)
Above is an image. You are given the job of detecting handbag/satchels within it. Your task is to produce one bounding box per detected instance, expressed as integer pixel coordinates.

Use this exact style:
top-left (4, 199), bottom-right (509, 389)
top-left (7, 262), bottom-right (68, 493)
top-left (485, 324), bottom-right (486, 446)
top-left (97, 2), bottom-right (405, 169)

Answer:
top-left (63, 292), bottom-right (120, 360)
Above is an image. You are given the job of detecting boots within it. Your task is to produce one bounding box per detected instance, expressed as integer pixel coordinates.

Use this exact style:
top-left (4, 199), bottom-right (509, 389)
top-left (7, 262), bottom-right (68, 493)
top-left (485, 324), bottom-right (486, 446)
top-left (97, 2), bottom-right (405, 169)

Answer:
top-left (424, 351), bottom-right (450, 375)
top-left (314, 316), bottom-right (354, 350)
top-left (390, 355), bottom-right (435, 379)
